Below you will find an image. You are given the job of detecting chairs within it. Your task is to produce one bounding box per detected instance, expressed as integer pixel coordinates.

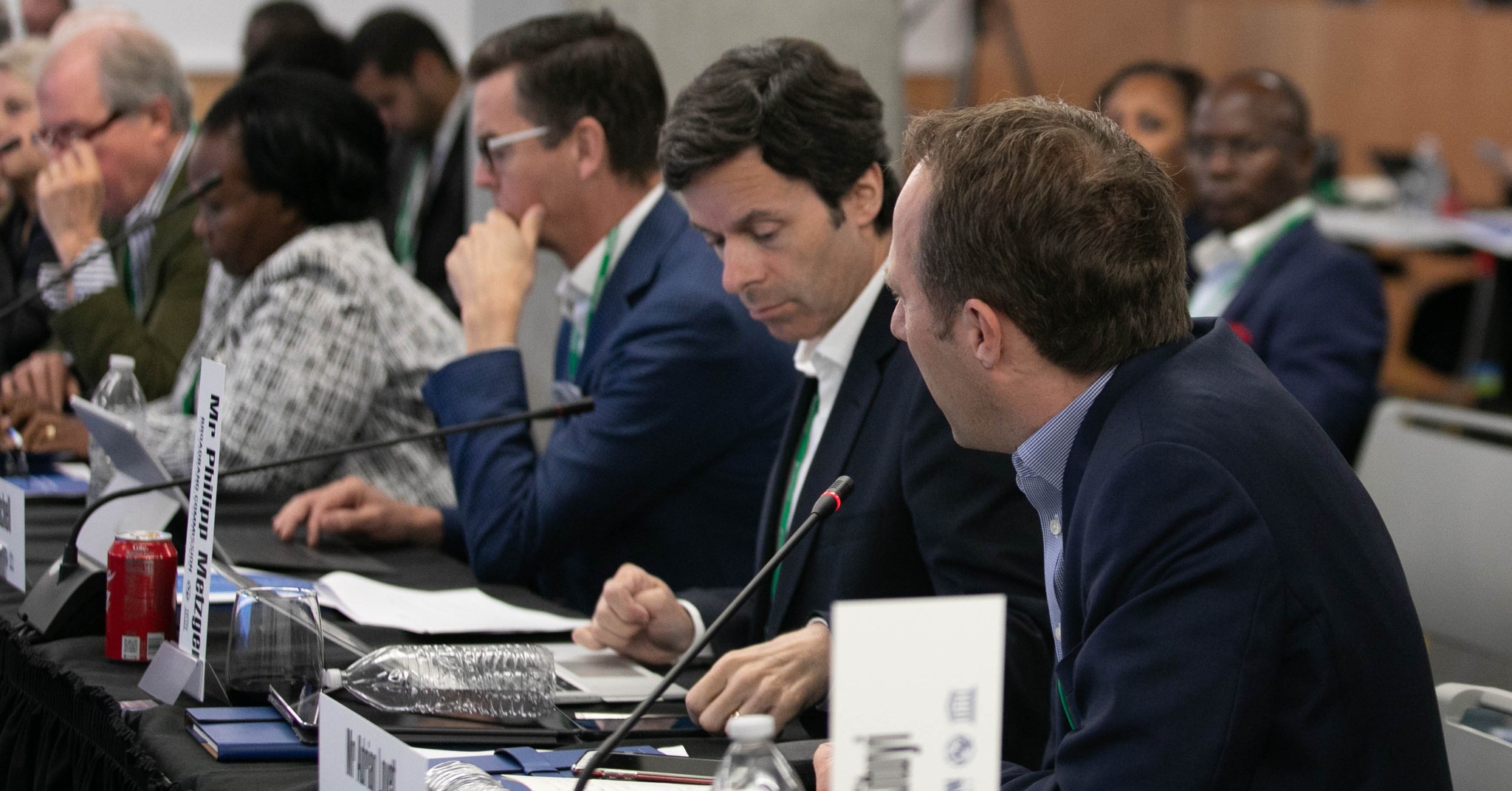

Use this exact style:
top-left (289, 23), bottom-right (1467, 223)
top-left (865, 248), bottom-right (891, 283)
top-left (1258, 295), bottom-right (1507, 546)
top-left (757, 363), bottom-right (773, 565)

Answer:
top-left (1345, 250), bottom-right (1511, 791)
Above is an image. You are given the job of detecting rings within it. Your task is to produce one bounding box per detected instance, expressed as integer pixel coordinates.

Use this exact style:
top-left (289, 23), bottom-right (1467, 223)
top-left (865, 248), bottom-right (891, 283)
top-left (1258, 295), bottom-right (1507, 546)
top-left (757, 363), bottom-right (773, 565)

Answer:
top-left (44, 423), bottom-right (57, 442)
top-left (734, 711), bottom-right (740, 717)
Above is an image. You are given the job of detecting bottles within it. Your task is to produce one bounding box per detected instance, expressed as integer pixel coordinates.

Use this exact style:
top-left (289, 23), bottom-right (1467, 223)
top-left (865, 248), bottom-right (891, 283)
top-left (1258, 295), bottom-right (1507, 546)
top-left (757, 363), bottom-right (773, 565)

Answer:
top-left (422, 760), bottom-right (510, 791)
top-left (323, 644), bottom-right (558, 717)
top-left (710, 712), bottom-right (805, 791)
top-left (87, 354), bottom-right (145, 508)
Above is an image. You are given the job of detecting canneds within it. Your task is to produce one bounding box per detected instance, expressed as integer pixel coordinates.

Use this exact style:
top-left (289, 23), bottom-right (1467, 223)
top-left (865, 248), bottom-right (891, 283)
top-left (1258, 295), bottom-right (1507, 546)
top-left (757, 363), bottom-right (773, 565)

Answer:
top-left (104, 529), bottom-right (177, 665)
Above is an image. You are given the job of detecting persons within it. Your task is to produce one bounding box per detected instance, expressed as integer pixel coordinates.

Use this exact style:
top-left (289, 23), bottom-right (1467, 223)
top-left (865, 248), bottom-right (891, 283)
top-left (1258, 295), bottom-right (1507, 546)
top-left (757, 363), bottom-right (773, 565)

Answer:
top-left (809, 78), bottom-right (1455, 791)
top-left (571, 31), bottom-right (1071, 769)
top-left (270, 5), bottom-right (800, 619)
top-left (0, 1), bottom-right (1188, 457)
top-left (1181, 68), bottom-right (1390, 473)
top-left (0, 21), bottom-right (217, 416)
top-left (0, 73), bottom-right (463, 519)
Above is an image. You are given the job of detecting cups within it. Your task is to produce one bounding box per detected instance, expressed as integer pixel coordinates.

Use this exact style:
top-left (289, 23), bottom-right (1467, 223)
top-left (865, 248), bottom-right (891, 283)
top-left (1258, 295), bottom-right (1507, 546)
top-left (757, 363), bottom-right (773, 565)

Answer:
top-left (225, 586), bottom-right (325, 706)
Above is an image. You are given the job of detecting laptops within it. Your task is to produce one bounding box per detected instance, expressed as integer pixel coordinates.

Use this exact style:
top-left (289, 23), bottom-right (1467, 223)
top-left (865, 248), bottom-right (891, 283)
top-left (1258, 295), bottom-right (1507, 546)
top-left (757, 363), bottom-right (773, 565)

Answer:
top-left (72, 396), bottom-right (398, 579)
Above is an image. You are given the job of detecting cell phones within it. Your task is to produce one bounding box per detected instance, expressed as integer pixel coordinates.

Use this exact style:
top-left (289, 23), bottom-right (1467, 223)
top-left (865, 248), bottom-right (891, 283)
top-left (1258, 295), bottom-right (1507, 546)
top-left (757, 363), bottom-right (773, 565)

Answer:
top-left (571, 750), bottom-right (724, 785)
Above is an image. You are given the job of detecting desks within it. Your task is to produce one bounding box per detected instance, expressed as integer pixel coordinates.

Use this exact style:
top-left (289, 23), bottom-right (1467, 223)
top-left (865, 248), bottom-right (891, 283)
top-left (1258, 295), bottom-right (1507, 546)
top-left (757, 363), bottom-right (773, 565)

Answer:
top-left (1, 448), bottom-right (833, 791)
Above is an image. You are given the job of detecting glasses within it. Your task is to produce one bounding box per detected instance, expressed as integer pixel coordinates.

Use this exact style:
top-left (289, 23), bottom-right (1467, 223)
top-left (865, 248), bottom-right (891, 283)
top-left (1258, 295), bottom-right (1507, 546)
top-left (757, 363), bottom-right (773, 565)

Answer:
top-left (29, 110), bottom-right (123, 154)
top-left (476, 126), bottom-right (552, 173)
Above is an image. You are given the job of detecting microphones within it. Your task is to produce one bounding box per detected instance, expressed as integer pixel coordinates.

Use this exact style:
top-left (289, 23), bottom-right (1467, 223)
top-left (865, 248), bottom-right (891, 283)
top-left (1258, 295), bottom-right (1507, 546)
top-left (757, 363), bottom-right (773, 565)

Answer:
top-left (0, 170), bottom-right (226, 321)
top-left (575, 473), bottom-right (855, 791)
top-left (20, 397), bottom-right (595, 638)
top-left (0, 135), bottom-right (21, 154)
